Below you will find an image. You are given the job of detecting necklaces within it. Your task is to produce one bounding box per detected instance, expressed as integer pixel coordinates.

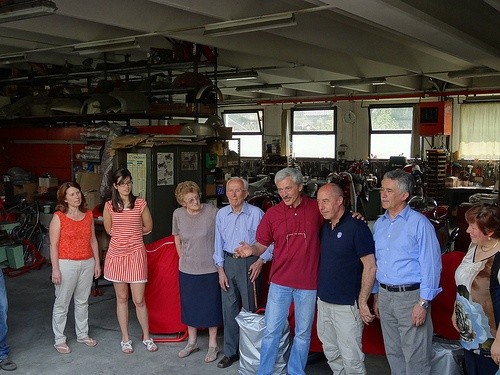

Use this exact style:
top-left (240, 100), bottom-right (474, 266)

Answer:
top-left (481, 239), bottom-right (498, 252)
top-left (329, 209), bottom-right (345, 226)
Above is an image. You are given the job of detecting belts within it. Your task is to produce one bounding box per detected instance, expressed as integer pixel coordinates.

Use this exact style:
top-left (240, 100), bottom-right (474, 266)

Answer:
top-left (224, 251), bottom-right (254, 259)
top-left (379, 282), bottom-right (421, 291)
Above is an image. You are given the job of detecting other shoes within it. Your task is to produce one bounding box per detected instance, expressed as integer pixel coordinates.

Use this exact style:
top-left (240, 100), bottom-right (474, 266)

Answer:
top-left (217, 352), bottom-right (240, 368)
top-left (0, 356), bottom-right (17, 371)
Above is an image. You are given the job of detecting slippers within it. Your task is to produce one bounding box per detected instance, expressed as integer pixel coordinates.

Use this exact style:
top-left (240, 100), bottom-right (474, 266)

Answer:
top-left (77, 336), bottom-right (97, 347)
top-left (53, 344), bottom-right (71, 354)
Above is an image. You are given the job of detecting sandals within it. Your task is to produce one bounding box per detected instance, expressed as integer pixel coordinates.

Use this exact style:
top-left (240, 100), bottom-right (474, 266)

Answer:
top-left (143, 338), bottom-right (158, 352)
top-left (120, 339), bottom-right (135, 353)
top-left (204, 346), bottom-right (221, 362)
top-left (178, 341), bottom-right (200, 358)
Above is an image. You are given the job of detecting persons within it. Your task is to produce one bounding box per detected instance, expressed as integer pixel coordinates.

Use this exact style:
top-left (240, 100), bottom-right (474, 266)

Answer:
top-left (49, 182), bottom-right (102, 354)
top-left (234, 167), bottom-right (366, 375)
top-left (370, 169), bottom-right (443, 375)
top-left (452, 203), bottom-right (500, 375)
top-left (317, 183), bottom-right (376, 375)
top-left (0, 268), bottom-right (17, 371)
top-left (212, 176), bottom-right (274, 368)
top-left (172, 181), bottom-right (222, 363)
top-left (103, 168), bottom-right (158, 354)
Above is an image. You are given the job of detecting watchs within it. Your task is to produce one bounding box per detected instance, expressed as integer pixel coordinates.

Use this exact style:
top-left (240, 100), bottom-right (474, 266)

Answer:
top-left (417, 299), bottom-right (430, 309)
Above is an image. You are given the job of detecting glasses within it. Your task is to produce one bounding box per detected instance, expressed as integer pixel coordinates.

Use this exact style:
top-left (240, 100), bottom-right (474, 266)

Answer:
top-left (379, 190), bottom-right (395, 195)
top-left (227, 188), bottom-right (247, 194)
top-left (117, 180), bottom-right (133, 187)
top-left (184, 192), bottom-right (202, 204)
